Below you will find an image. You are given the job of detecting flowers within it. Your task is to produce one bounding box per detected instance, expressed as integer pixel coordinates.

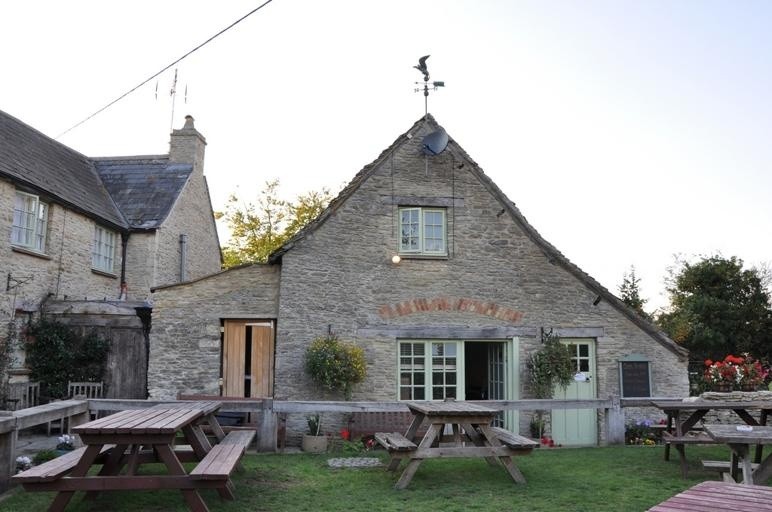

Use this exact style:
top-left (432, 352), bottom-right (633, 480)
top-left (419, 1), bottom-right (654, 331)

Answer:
top-left (703, 354), bottom-right (769, 393)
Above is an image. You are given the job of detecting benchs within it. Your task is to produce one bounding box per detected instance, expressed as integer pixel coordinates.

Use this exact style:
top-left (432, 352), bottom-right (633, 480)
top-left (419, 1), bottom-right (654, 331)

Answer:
top-left (643, 399), bottom-right (771, 512)
top-left (375, 402), bottom-right (539, 491)
top-left (11, 397), bottom-right (258, 512)
